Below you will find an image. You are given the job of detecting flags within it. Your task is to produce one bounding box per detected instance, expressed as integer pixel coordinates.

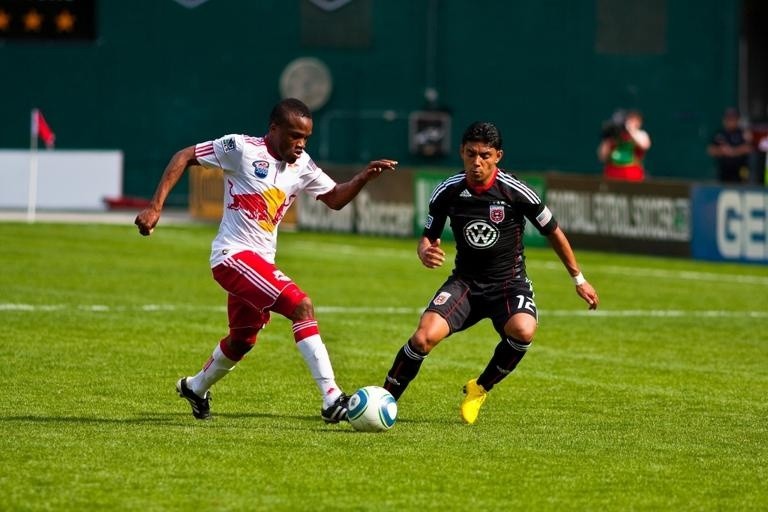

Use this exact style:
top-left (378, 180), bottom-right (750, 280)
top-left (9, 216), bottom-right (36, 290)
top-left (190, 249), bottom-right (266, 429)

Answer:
top-left (37, 111), bottom-right (56, 149)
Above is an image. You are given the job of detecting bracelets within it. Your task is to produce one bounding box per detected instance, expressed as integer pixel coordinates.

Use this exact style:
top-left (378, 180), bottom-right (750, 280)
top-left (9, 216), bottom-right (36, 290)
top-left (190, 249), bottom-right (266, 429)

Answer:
top-left (569, 271), bottom-right (586, 285)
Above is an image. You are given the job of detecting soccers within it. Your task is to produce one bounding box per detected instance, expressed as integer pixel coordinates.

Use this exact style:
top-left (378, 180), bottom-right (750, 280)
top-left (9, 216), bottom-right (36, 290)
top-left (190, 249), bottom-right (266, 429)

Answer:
top-left (347, 385), bottom-right (397, 431)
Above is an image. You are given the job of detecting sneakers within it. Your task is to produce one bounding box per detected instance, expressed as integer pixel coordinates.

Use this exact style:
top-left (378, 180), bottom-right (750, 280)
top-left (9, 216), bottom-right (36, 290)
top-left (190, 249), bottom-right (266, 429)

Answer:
top-left (175, 375), bottom-right (212, 419)
top-left (320, 391), bottom-right (353, 424)
top-left (461, 378), bottom-right (489, 426)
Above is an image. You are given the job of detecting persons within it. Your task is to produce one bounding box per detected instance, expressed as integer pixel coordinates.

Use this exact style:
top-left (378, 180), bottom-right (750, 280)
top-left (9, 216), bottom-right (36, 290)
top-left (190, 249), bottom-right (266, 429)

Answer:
top-left (596, 109), bottom-right (653, 182)
top-left (705, 106), bottom-right (751, 182)
top-left (416, 85), bottom-right (457, 164)
top-left (134, 95), bottom-right (401, 424)
top-left (381, 119), bottom-right (600, 426)
